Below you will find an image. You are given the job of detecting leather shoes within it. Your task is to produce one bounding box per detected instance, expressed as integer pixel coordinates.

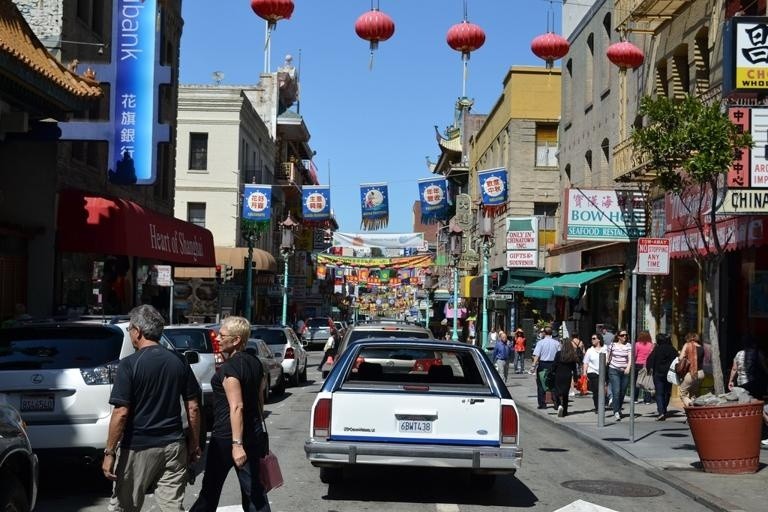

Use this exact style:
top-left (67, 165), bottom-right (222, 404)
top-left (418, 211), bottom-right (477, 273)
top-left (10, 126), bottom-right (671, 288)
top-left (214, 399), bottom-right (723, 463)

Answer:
top-left (656, 414), bottom-right (665, 421)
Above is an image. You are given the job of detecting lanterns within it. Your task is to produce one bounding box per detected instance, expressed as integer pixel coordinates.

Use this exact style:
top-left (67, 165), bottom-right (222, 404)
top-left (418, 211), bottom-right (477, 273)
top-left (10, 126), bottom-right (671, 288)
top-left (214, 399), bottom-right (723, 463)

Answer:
top-left (605, 42), bottom-right (646, 76)
top-left (531, 31), bottom-right (572, 62)
top-left (251, 0), bottom-right (295, 23)
top-left (446, 20), bottom-right (488, 53)
top-left (355, 7), bottom-right (396, 40)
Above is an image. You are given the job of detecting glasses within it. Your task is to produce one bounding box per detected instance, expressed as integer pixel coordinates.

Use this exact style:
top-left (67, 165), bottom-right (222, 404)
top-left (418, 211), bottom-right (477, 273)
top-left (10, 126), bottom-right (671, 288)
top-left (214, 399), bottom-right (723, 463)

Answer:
top-left (619, 334), bottom-right (628, 337)
top-left (218, 331), bottom-right (241, 339)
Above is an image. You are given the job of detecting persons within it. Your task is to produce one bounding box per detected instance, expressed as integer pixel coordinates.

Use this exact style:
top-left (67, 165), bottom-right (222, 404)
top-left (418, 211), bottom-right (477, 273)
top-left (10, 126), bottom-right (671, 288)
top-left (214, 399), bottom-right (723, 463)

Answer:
top-left (465, 320), bottom-right (477, 346)
top-left (276, 316), bottom-right (305, 340)
top-left (317, 331), bottom-right (336, 369)
top-left (337, 328), bottom-right (346, 350)
top-left (529, 325), bottom-right (714, 426)
top-left (101, 304), bottom-right (201, 512)
top-left (187, 315), bottom-right (272, 512)
top-left (761, 404), bottom-right (768, 446)
top-left (487, 325), bottom-right (527, 385)
top-left (728, 335), bottom-right (768, 402)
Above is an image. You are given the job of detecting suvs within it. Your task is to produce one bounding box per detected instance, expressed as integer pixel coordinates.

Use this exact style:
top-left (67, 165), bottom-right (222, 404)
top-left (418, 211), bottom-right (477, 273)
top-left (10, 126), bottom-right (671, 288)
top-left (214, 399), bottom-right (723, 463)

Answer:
top-left (247, 317), bottom-right (348, 387)
top-left (163, 321), bottom-right (233, 415)
top-left (0, 309), bottom-right (208, 493)
top-left (332, 321), bottom-right (442, 381)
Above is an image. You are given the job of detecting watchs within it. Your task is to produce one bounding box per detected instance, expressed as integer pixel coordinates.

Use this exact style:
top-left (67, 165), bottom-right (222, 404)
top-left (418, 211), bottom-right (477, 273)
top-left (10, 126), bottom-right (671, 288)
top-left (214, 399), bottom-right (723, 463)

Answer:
top-left (103, 448), bottom-right (115, 455)
top-left (232, 440), bottom-right (243, 444)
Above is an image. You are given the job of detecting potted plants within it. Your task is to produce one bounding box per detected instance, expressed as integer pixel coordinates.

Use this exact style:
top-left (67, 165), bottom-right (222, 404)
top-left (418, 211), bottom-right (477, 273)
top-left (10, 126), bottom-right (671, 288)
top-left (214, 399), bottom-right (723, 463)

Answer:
top-left (622, 77), bottom-right (768, 479)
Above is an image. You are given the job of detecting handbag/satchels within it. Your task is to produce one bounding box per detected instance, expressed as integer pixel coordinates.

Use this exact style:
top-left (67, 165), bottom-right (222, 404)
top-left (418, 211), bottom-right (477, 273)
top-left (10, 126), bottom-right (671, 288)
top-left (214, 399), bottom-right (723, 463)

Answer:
top-left (260, 431), bottom-right (283, 491)
top-left (675, 358), bottom-right (691, 376)
top-left (605, 362), bottom-right (609, 385)
top-left (667, 357), bottom-right (681, 386)
top-left (636, 368), bottom-right (656, 390)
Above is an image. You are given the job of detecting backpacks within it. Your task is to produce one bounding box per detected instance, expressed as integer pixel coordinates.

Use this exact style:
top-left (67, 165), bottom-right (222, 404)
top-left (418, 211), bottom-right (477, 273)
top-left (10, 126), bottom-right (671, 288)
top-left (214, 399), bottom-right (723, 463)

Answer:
top-left (572, 340), bottom-right (583, 363)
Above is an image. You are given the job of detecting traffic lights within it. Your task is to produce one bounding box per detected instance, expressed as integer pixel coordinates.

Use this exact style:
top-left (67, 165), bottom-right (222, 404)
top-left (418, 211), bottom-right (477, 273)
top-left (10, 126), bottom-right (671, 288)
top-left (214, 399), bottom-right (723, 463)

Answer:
top-left (214, 265), bottom-right (222, 285)
top-left (220, 263), bottom-right (232, 285)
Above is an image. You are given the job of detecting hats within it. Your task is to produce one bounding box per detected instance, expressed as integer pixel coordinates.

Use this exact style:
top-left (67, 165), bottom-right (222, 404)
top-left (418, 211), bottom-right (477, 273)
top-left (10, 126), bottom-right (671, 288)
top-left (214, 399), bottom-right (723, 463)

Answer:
top-left (516, 328), bottom-right (524, 334)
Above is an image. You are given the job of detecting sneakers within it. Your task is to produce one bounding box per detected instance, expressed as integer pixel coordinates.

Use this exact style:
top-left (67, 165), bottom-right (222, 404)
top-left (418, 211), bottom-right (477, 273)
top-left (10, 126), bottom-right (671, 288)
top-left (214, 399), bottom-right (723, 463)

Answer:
top-left (538, 395), bottom-right (568, 417)
top-left (609, 398), bottom-right (623, 420)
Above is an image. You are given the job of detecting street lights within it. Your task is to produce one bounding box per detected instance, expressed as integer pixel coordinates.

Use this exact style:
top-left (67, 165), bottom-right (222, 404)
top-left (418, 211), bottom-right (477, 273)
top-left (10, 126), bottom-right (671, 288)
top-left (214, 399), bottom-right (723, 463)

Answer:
top-left (242, 228), bottom-right (260, 324)
top-left (275, 208), bottom-right (300, 330)
top-left (474, 203), bottom-right (495, 356)
top-left (444, 235), bottom-right (465, 339)
top-left (422, 271), bottom-right (433, 327)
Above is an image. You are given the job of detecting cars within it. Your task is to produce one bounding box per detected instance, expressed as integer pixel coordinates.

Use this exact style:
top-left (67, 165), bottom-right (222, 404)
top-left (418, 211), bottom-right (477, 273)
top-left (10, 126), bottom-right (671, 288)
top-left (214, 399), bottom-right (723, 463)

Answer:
top-left (0, 396), bottom-right (40, 510)
top-left (244, 338), bottom-right (287, 402)
top-left (300, 333), bottom-right (524, 494)
